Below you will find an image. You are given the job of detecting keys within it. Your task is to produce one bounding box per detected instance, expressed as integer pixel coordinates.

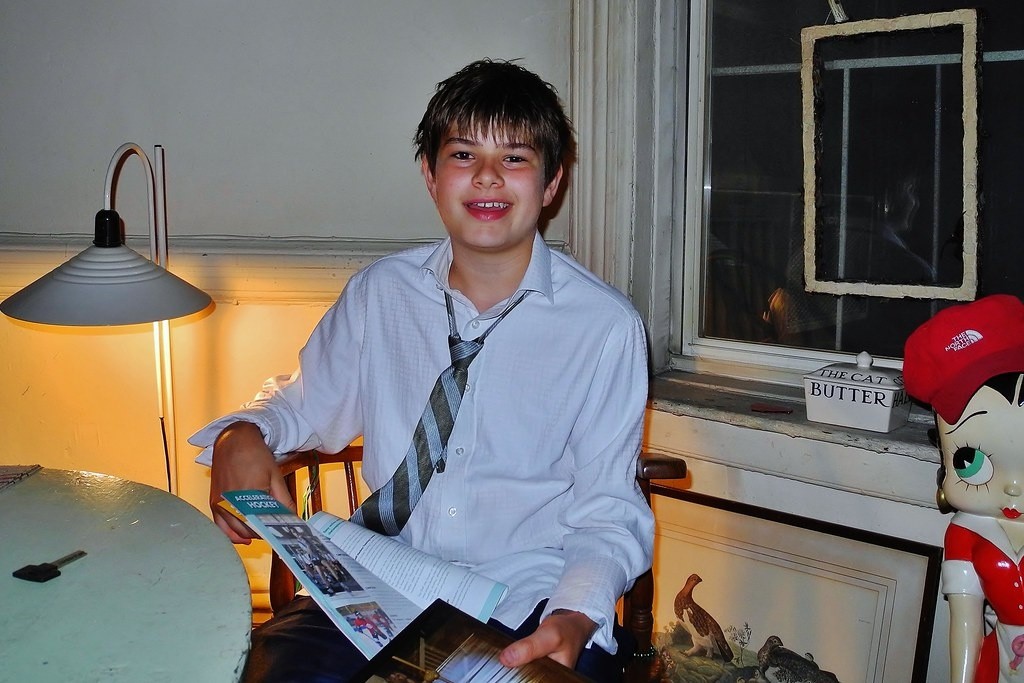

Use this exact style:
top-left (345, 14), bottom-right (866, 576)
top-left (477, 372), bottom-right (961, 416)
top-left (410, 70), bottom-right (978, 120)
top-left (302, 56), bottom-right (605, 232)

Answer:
top-left (12, 550), bottom-right (87, 583)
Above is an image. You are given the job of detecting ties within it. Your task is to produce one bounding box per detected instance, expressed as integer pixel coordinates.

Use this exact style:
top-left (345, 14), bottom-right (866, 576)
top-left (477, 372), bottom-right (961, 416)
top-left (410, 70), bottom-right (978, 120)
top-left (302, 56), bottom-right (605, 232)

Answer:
top-left (348, 291), bottom-right (536, 537)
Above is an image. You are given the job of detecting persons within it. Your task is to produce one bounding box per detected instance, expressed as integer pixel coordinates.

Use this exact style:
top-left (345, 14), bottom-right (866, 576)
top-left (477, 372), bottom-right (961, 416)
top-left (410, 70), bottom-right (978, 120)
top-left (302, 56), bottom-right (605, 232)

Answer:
top-left (903, 295), bottom-right (1023, 683)
top-left (210, 61), bottom-right (656, 683)
top-left (769, 158), bottom-right (937, 347)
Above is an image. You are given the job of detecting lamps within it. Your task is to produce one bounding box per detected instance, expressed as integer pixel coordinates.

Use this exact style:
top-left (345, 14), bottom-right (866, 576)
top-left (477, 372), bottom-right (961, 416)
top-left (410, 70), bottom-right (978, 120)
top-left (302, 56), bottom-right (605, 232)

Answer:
top-left (0, 141), bottom-right (213, 497)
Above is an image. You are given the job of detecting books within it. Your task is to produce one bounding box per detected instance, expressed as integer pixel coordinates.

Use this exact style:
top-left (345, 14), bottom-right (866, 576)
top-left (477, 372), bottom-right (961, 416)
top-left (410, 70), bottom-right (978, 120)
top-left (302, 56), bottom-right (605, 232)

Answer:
top-left (217, 490), bottom-right (589, 683)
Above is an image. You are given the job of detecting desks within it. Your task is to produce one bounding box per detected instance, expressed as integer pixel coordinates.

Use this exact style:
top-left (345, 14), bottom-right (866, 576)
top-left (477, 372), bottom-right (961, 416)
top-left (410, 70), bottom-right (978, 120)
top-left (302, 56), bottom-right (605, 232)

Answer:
top-left (0, 468), bottom-right (252, 683)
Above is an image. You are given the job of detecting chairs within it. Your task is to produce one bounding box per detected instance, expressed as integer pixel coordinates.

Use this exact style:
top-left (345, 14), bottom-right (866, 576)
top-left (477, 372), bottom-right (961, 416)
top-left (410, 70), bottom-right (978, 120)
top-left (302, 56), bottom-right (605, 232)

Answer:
top-left (269, 446), bottom-right (686, 683)
